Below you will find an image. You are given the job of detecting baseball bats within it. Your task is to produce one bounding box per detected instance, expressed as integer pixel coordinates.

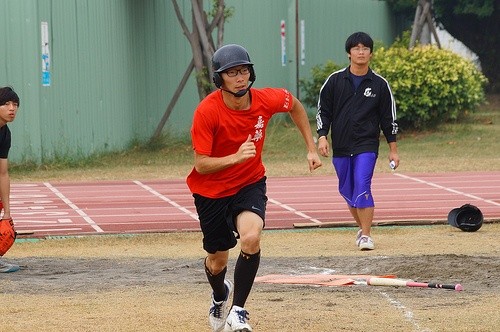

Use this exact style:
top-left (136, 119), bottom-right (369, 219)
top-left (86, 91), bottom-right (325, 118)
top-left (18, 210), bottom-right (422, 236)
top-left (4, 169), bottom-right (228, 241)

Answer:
top-left (367, 277), bottom-right (463, 292)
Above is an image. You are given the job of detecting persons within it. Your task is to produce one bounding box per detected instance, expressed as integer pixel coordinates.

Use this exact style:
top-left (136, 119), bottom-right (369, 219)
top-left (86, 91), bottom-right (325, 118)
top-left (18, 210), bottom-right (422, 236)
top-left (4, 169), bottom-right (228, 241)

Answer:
top-left (185, 45), bottom-right (322, 332)
top-left (0, 87), bottom-right (21, 272)
top-left (315, 32), bottom-right (400, 250)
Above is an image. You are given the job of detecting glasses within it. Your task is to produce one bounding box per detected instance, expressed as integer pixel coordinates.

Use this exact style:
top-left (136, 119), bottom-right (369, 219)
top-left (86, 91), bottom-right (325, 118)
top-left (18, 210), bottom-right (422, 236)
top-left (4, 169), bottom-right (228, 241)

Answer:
top-left (221, 66), bottom-right (251, 77)
top-left (351, 47), bottom-right (370, 51)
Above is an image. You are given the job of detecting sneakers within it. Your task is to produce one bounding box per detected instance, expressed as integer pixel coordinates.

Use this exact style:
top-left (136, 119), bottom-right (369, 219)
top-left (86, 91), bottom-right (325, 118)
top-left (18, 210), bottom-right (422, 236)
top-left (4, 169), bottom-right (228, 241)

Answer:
top-left (356, 229), bottom-right (374, 250)
top-left (208, 279), bottom-right (234, 332)
top-left (224, 305), bottom-right (253, 332)
top-left (0, 258), bottom-right (20, 272)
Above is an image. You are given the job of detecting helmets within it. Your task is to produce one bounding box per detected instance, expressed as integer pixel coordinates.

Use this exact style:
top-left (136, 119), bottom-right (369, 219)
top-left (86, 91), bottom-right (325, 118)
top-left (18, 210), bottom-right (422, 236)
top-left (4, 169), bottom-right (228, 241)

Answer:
top-left (447, 204), bottom-right (483, 232)
top-left (211, 43), bottom-right (256, 97)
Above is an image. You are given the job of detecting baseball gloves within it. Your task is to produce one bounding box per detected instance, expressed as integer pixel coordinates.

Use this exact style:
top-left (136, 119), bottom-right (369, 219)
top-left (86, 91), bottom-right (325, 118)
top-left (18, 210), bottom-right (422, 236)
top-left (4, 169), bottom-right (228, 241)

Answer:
top-left (0, 218), bottom-right (18, 256)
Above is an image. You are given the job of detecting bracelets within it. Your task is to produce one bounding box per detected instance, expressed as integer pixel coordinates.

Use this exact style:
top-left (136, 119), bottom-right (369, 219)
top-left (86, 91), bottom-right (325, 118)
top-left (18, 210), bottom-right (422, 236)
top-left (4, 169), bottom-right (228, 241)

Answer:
top-left (318, 136), bottom-right (326, 140)
